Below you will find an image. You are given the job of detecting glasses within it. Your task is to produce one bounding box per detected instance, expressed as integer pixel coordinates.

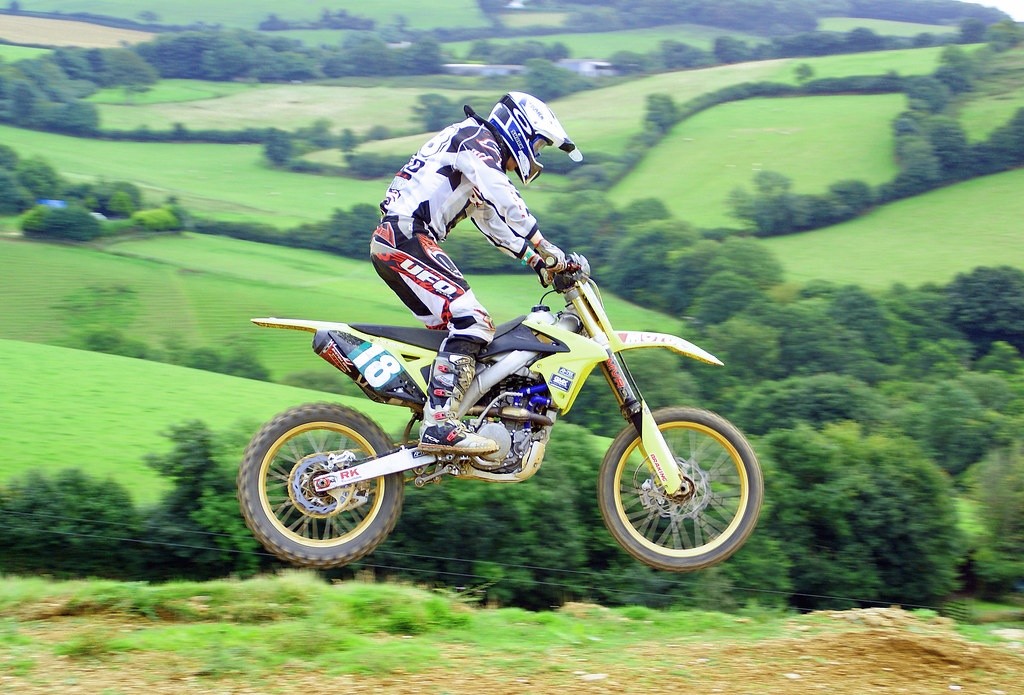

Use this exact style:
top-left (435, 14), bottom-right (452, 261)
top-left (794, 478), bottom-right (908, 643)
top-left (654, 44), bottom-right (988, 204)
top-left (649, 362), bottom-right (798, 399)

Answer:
top-left (499, 96), bottom-right (545, 159)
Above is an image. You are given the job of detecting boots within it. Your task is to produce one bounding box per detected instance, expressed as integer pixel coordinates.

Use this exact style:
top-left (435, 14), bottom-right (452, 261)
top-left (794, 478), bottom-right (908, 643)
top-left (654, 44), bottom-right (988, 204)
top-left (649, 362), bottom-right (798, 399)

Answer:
top-left (418, 351), bottom-right (500, 455)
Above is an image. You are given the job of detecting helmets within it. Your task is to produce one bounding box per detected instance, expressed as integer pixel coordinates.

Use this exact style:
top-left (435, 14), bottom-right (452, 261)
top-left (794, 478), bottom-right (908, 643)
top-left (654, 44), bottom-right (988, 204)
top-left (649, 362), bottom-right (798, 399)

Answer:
top-left (488, 92), bottom-right (583, 187)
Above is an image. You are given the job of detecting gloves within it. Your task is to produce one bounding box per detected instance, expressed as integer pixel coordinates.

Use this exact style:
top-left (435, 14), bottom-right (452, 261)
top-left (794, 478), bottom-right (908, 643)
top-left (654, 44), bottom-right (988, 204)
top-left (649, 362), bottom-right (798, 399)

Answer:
top-left (535, 239), bottom-right (567, 273)
top-left (527, 256), bottom-right (555, 288)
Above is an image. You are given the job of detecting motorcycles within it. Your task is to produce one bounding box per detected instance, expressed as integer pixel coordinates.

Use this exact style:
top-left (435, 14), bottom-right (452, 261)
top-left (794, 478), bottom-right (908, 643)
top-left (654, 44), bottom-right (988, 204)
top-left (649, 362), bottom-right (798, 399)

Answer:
top-left (237, 253), bottom-right (766, 571)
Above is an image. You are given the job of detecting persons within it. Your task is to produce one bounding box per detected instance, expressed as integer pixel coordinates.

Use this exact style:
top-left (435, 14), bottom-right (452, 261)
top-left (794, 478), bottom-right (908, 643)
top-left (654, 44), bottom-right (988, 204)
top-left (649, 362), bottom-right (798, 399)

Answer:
top-left (371, 92), bottom-right (583, 454)
